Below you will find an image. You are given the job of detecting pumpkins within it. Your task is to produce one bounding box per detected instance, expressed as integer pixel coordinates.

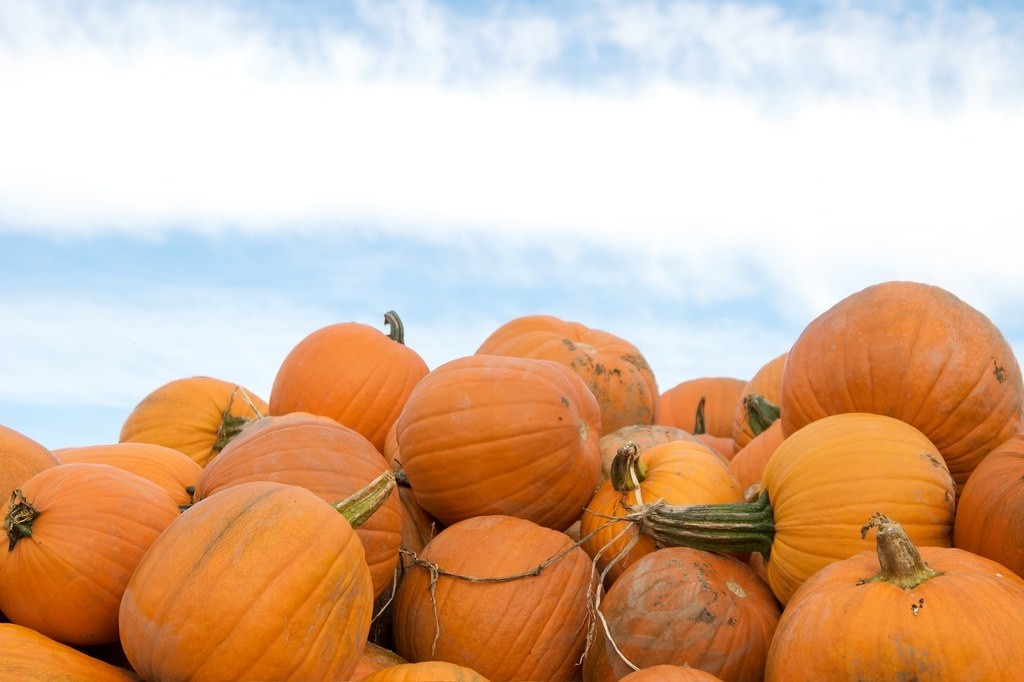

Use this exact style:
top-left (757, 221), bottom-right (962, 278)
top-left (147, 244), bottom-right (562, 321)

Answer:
top-left (0, 281), bottom-right (1024, 682)
